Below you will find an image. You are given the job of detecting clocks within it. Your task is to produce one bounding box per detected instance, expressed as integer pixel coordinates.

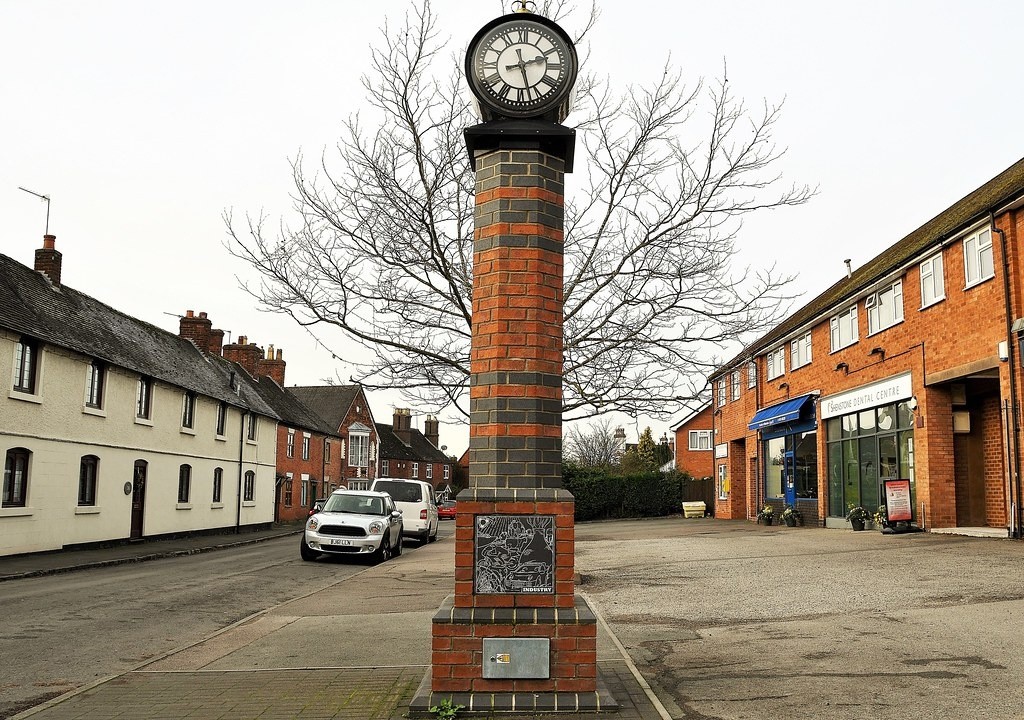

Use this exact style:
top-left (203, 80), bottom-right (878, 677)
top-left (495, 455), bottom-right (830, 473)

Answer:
top-left (465, 11), bottom-right (578, 119)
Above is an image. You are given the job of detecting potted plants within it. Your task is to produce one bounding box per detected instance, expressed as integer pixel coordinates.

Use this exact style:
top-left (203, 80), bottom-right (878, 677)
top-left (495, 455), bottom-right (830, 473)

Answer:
top-left (845, 506), bottom-right (866, 531)
top-left (757, 503), bottom-right (774, 526)
top-left (859, 508), bottom-right (875, 530)
top-left (780, 505), bottom-right (801, 527)
top-left (874, 505), bottom-right (889, 528)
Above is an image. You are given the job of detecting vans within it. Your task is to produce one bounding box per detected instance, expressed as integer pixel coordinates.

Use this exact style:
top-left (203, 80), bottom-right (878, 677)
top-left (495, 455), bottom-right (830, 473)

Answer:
top-left (370, 478), bottom-right (439, 546)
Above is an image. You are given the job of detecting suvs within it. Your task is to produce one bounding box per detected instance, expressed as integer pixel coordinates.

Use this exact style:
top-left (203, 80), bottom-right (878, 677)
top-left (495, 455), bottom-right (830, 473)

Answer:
top-left (299, 486), bottom-right (404, 566)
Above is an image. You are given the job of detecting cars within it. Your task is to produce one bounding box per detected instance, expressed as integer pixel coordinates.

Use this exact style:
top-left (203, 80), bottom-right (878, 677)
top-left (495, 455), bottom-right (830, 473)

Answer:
top-left (304, 497), bottom-right (327, 526)
top-left (436, 500), bottom-right (457, 521)
